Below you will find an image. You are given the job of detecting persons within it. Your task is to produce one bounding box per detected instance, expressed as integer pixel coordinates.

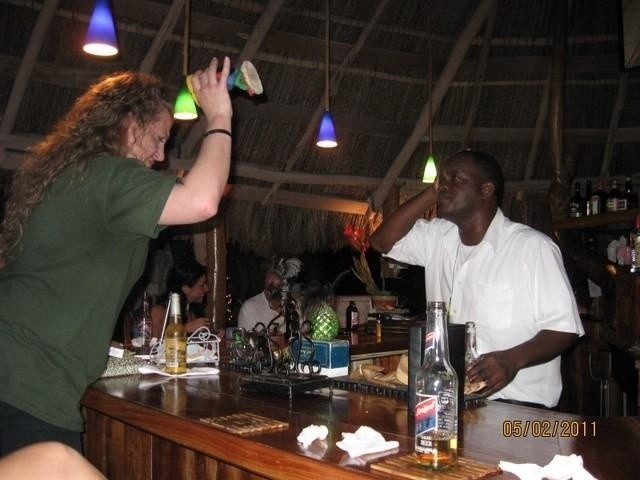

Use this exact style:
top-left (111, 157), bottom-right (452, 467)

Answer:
top-left (237, 273), bottom-right (305, 349)
top-left (130, 262), bottom-right (224, 354)
top-left (368, 151), bottom-right (586, 412)
top-left (0, 56), bottom-right (233, 480)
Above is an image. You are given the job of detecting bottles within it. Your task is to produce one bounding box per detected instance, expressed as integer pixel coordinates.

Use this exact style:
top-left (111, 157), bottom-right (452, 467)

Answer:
top-left (570, 181), bottom-right (582, 219)
top-left (463, 320), bottom-right (481, 397)
top-left (620, 175), bottom-right (634, 211)
top-left (164, 290), bottom-right (190, 374)
top-left (592, 180), bottom-right (607, 216)
top-left (607, 177), bottom-right (620, 213)
top-left (583, 179), bottom-right (592, 217)
top-left (345, 298), bottom-right (362, 335)
top-left (375, 310), bottom-right (384, 343)
top-left (413, 300), bottom-right (459, 457)
top-left (131, 285), bottom-right (155, 358)
top-left (286, 302), bottom-right (300, 345)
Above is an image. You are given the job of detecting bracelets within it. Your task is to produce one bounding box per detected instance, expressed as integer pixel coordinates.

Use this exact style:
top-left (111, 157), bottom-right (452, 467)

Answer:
top-left (203, 129), bottom-right (232, 138)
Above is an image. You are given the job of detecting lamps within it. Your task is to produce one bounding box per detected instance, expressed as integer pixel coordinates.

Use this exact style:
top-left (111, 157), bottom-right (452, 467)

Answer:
top-left (82, 0), bottom-right (119, 57)
top-left (422, 0), bottom-right (438, 185)
top-left (316, 1), bottom-right (338, 148)
top-left (173, 0), bottom-right (198, 121)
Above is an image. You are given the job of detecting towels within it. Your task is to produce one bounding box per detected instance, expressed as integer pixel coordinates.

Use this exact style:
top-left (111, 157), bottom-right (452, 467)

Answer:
top-left (296, 424), bottom-right (328, 449)
top-left (335, 425), bottom-right (401, 459)
top-left (139, 364), bottom-right (221, 380)
top-left (498, 453), bottom-right (597, 480)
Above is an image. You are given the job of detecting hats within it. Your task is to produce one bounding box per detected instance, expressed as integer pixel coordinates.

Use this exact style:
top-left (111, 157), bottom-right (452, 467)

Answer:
top-left (268, 252), bottom-right (304, 283)
